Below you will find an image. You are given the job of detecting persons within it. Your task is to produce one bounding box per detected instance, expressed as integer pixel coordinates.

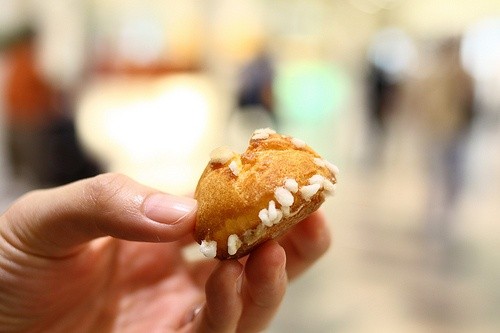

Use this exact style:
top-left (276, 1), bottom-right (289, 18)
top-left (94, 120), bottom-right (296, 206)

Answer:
top-left (3, 17), bottom-right (478, 267)
top-left (1, 171), bottom-right (332, 332)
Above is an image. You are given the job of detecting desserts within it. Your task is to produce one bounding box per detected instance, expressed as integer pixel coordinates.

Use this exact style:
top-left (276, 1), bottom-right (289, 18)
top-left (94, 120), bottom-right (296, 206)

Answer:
top-left (191, 128), bottom-right (338, 261)
